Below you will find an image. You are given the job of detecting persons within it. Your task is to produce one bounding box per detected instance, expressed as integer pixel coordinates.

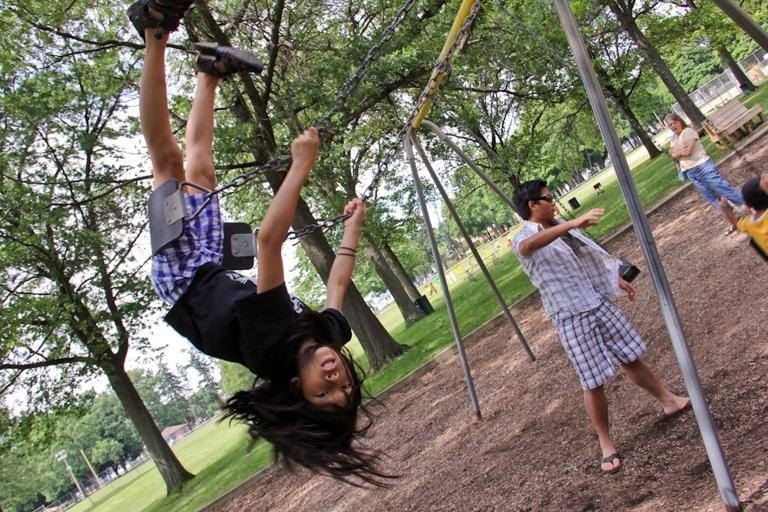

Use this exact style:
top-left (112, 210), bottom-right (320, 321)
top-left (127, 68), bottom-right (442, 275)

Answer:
top-left (510, 177), bottom-right (695, 474)
top-left (716, 171), bottom-right (766, 256)
top-left (663, 112), bottom-right (749, 236)
top-left (125, 0), bottom-right (404, 497)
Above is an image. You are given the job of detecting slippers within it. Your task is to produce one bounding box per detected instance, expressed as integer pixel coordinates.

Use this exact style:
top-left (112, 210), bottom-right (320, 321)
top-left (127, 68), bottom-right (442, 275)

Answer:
top-left (665, 397), bottom-right (710, 416)
top-left (601, 453), bottom-right (620, 473)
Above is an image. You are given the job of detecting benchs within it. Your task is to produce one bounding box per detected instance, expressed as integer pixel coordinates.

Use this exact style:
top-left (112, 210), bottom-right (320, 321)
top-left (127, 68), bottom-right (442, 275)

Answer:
top-left (700, 98), bottom-right (767, 148)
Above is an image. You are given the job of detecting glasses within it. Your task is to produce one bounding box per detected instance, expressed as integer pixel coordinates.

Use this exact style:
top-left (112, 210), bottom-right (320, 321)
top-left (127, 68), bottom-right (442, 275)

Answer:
top-left (534, 195), bottom-right (552, 202)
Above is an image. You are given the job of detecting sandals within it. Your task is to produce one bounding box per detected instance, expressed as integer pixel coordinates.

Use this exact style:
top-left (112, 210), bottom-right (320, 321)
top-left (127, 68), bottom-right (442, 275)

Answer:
top-left (193, 42), bottom-right (263, 78)
top-left (126, 0), bottom-right (193, 42)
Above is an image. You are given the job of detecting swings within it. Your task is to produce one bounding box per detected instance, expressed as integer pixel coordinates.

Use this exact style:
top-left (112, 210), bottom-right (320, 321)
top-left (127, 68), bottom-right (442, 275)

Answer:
top-left (433, 88), bottom-right (641, 283)
top-left (148, 1), bottom-right (481, 270)
top-left (496, 2), bottom-right (768, 262)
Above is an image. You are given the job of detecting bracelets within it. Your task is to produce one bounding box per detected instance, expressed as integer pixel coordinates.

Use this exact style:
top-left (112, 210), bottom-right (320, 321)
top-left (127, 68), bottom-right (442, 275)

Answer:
top-left (338, 246), bottom-right (356, 253)
top-left (336, 251), bottom-right (356, 258)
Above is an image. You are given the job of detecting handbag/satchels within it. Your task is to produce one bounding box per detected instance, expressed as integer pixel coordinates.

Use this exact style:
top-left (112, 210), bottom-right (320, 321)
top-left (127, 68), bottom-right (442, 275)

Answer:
top-left (678, 170), bottom-right (686, 183)
